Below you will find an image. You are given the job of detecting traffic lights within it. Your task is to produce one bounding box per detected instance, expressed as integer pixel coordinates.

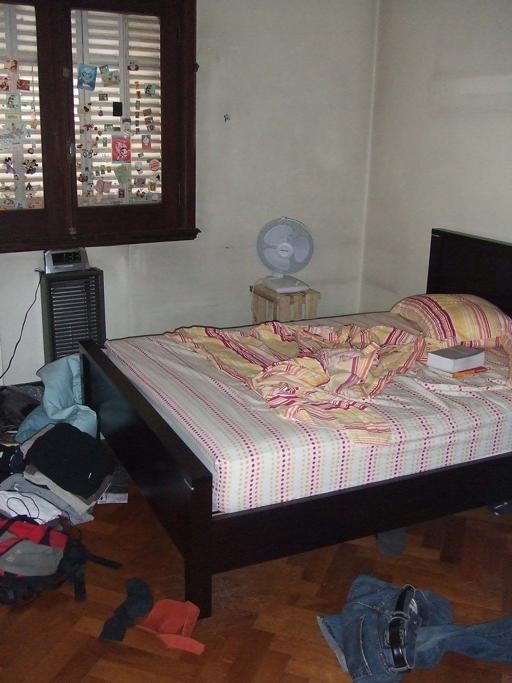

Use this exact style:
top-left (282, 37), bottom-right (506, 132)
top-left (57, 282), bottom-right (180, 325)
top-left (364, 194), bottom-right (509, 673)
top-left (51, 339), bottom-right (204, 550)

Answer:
top-left (391, 293), bottom-right (511, 349)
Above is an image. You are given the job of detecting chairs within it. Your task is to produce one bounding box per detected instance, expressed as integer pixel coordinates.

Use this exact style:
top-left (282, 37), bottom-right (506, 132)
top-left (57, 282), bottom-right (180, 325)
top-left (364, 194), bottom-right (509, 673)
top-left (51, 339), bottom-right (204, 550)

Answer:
top-left (43, 517), bottom-right (82, 540)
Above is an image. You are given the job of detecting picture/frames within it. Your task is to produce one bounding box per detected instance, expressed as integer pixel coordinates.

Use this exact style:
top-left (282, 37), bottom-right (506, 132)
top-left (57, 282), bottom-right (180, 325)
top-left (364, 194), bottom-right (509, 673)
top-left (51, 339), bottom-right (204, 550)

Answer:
top-left (250, 285), bottom-right (321, 324)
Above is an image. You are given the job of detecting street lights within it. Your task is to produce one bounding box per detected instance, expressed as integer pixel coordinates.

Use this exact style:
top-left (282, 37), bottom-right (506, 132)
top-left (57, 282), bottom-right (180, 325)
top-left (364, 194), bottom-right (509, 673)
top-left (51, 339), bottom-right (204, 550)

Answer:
top-left (1, 515), bottom-right (68, 578)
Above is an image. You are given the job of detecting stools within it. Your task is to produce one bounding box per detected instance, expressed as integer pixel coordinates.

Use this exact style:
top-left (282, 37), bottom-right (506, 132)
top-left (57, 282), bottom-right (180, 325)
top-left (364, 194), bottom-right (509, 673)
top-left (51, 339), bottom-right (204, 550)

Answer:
top-left (387, 586), bottom-right (409, 674)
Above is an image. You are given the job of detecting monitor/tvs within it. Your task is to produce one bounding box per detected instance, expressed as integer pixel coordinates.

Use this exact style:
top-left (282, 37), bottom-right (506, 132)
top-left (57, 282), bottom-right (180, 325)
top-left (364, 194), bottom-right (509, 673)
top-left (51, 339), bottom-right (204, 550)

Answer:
top-left (426, 345), bottom-right (485, 374)
top-left (428, 365), bottom-right (488, 381)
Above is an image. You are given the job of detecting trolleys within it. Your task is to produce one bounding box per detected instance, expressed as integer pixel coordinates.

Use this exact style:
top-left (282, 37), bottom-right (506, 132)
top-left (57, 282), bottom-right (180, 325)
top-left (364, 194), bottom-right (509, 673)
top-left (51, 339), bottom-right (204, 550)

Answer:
top-left (257, 217), bottom-right (314, 293)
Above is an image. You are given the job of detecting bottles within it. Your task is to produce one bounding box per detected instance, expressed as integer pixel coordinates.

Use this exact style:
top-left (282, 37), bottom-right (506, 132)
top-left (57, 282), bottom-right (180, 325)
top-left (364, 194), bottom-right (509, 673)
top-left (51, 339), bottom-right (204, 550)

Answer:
top-left (137, 597), bottom-right (205, 656)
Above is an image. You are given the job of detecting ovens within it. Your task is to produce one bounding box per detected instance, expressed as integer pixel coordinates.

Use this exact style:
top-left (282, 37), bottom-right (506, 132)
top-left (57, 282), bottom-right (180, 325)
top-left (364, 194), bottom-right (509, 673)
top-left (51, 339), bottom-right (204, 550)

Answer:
top-left (77, 227), bottom-right (511, 619)
